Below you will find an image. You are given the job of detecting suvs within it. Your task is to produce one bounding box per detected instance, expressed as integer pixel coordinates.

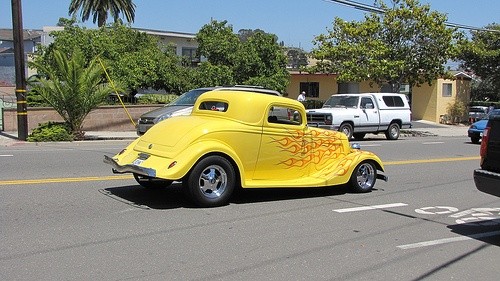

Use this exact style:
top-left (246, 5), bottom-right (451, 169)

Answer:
top-left (294, 94), bottom-right (413, 140)
top-left (136, 86), bottom-right (284, 138)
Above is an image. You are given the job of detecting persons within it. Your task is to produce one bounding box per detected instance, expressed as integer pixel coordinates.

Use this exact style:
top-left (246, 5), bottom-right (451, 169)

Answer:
top-left (297, 91), bottom-right (306, 102)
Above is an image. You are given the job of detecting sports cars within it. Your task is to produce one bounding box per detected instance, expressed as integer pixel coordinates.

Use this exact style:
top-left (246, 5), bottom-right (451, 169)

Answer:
top-left (103, 88), bottom-right (390, 206)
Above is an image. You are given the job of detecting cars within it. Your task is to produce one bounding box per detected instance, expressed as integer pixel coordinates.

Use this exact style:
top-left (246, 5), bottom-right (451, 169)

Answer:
top-left (469, 105), bottom-right (488, 120)
top-left (468, 108), bottom-right (499, 143)
